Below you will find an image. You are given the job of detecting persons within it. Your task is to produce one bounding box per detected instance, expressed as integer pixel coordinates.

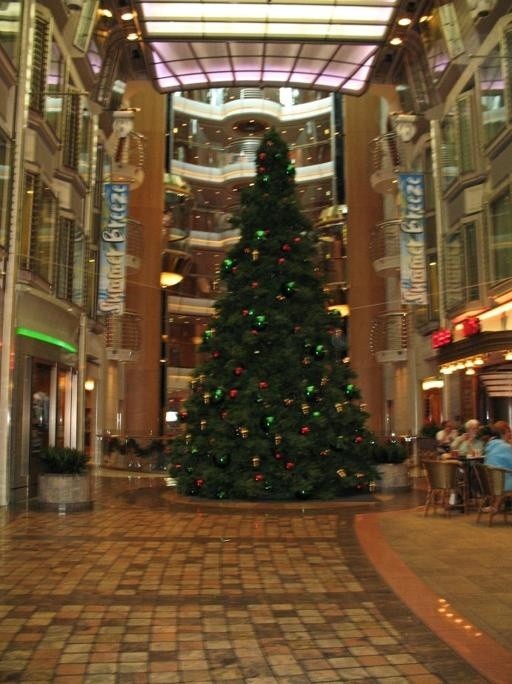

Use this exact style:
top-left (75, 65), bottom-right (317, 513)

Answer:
top-left (435, 418), bottom-right (511, 512)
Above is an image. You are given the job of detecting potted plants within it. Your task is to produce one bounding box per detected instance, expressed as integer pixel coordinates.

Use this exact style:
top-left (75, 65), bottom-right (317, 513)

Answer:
top-left (373, 442), bottom-right (420, 495)
top-left (32, 443), bottom-right (95, 506)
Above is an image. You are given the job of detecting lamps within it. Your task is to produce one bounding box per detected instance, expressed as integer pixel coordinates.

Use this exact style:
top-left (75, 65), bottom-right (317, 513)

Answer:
top-left (159, 248), bottom-right (196, 291)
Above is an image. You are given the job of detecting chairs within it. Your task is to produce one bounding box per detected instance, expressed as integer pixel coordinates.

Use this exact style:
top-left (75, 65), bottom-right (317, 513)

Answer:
top-left (420, 453), bottom-right (512, 528)
